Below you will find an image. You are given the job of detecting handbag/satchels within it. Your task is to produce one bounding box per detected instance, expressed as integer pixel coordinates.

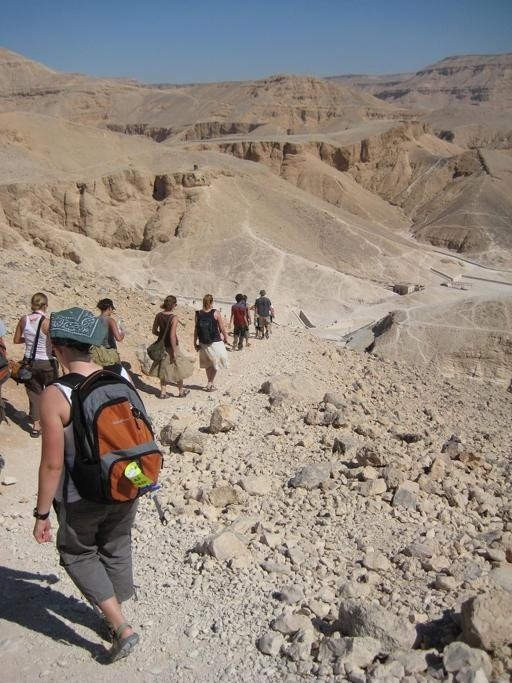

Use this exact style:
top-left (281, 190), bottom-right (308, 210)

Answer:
top-left (0, 344), bottom-right (10, 386)
top-left (11, 359), bottom-right (34, 386)
top-left (147, 339), bottom-right (166, 362)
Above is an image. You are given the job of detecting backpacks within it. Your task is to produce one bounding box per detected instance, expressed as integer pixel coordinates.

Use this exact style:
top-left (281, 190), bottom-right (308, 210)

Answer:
top-left (198, 309), bottom-right (217, 345)
top-left (46, 363), bottom-right (164, 506)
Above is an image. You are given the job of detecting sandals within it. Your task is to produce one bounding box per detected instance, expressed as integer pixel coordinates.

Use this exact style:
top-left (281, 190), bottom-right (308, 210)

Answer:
top-left (207, 385), bottom-right (218, 392)
top-left (179, 390), bottom-right (191, 398)
top-left (109, 623), bottom-right (140, 664)
top-left (159, 393), bottom-right (170, 398)
top-left (30, 428), bottom-right (43, 438)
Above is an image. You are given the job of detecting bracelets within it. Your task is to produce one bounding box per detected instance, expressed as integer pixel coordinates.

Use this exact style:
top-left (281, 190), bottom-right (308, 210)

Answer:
top-left (34, 507), bottom-right (50, 520)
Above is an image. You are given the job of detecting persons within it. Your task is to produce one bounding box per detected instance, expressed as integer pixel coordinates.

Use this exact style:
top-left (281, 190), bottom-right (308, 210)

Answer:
top-left (14, 292), bottom-right (58, 438)
top-left (228, 289), bottom-right (275, 351)
top-left (34, 305), bottom-right (139, 663)
top-left (193, 294), bottom-right (227, 391)
top-left (92, 297), bottom-right (126, 368)
top-left (1, 319), bottom-right (8, 425)
top-left (150, 294), bottom-right (195, 400)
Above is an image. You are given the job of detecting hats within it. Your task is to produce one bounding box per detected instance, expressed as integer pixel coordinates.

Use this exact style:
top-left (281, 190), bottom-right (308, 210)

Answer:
top-left (104, 298), bottom-right (116, 310)
